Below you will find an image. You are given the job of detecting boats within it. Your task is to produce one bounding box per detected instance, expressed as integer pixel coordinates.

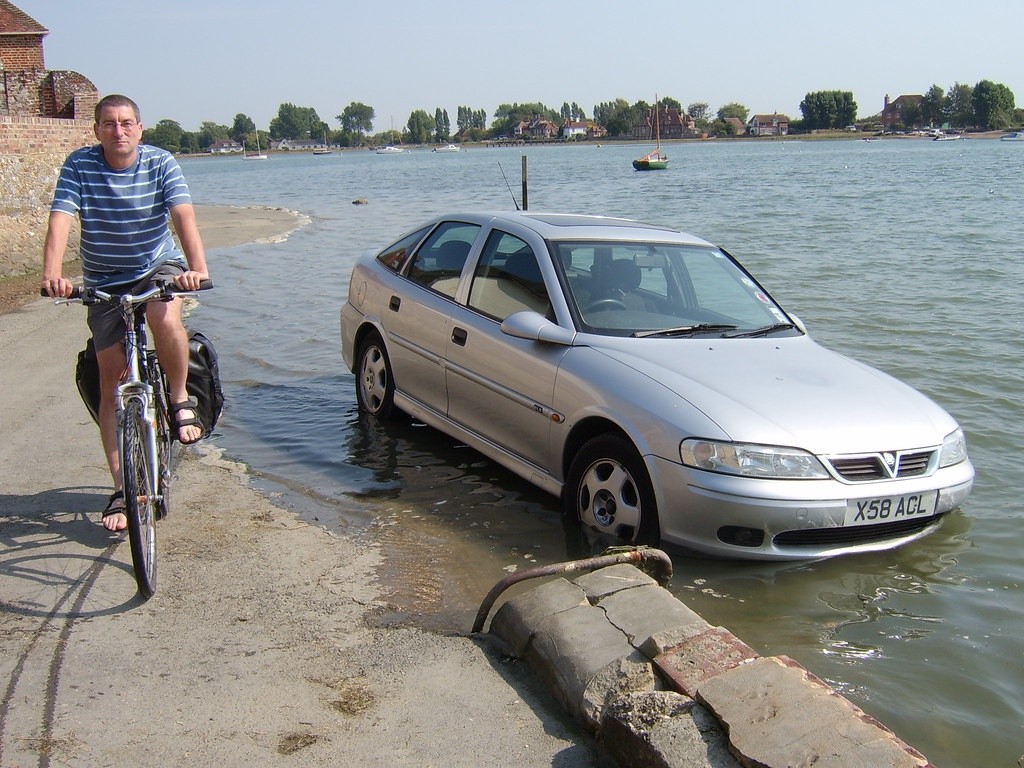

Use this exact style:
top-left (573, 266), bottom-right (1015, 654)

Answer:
top-left (432, 143), bottom-right (460, 152)
top-left (377, 145), bottom-right (405, 154)
top-left (933, 135), bottom-right (961, 141)
top-left (1000, 130), bottom-right (1024, 142)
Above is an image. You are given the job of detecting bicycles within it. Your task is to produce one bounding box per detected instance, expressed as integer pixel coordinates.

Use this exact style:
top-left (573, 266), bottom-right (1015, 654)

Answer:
top-left (37, 278), bottom-right (215, 597)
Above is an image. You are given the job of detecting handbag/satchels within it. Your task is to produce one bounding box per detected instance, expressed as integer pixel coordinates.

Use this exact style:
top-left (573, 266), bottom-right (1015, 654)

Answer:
top-left (75, 326), bottom-right (224, 438)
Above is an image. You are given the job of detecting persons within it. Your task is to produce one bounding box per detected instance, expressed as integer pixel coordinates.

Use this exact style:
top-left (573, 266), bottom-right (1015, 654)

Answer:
top-left (42, 95), bottom-right (209, 531)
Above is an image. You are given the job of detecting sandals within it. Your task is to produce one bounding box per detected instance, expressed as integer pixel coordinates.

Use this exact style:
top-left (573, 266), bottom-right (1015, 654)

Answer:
top-left (168, 394), bottom-right (205, 445)
top-left (102, 491), bottom-right (128, 532)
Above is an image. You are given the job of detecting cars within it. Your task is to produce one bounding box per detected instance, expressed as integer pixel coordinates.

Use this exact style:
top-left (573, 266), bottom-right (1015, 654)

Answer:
top-left (338, 213), bottom-right (978, 565)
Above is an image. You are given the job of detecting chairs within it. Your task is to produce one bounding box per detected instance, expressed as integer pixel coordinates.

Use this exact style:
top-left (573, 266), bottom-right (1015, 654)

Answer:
top-left (426, 240), bottom-right (582, 321)
top-left (589, 259), bottom-right (659, 312)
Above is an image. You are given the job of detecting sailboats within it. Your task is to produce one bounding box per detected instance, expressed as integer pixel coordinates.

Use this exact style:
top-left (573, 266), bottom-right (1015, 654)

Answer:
top-left (312, 130), bottom-right (333, 155)
top-left (632, 93), bottom-right (671, 170)
top-left (241, 123), bottom-right (268, 159)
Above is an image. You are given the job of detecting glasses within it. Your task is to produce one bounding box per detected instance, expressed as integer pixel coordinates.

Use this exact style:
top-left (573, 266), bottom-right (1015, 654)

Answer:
top-left (99, 121), bottom-right (138, 132)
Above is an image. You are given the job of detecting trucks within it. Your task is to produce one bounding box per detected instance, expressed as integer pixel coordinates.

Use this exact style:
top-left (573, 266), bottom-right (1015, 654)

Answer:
top-left (928, 128), bottom-right (944, 137)
